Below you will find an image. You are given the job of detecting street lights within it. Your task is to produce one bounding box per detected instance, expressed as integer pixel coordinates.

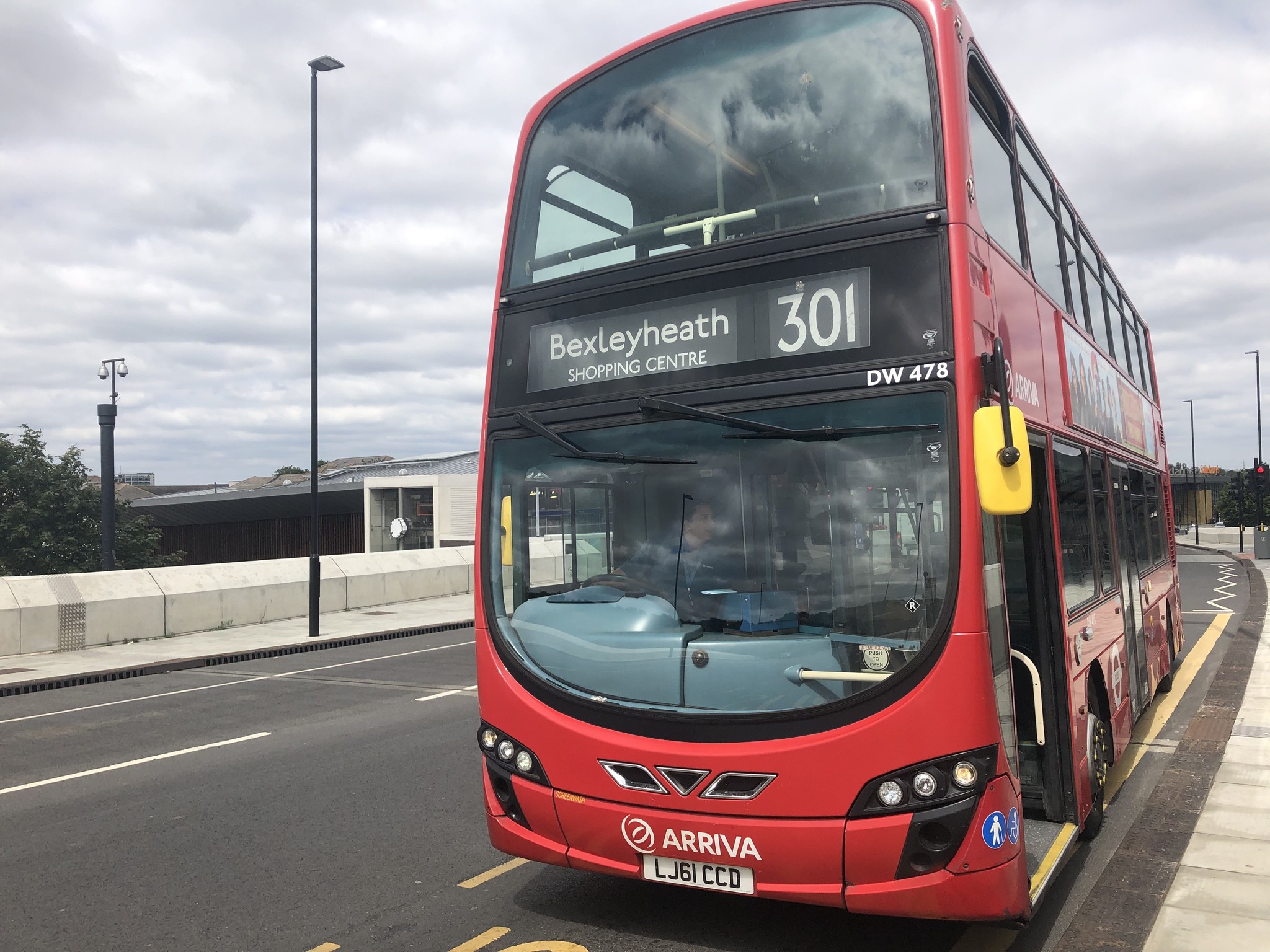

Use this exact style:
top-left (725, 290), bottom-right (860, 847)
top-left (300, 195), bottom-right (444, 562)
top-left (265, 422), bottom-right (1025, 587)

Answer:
top-left (1182, 399), bottom-right (1199, 545)
top-left (307, 56), bottom-right (345, 637)
top-left (1184, 468), bottom-right (1189, 531)
top-left (1245, 349), bottom-right (1263, 532)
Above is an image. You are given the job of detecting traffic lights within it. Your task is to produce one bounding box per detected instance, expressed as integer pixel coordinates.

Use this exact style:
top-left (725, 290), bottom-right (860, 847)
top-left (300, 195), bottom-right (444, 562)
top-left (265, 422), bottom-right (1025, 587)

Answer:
top-left (1255, 465), bottom-right (1270, 496)
top-left (1244, 467), bottom-right (1255, 493)
top-left (1230, 477), bottom-right (1243, 499)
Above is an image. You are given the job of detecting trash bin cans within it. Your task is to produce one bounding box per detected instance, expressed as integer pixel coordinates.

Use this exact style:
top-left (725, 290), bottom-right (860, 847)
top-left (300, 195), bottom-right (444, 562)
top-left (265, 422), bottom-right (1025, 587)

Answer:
top-left (1254, 525), bottom-right (1270, 559)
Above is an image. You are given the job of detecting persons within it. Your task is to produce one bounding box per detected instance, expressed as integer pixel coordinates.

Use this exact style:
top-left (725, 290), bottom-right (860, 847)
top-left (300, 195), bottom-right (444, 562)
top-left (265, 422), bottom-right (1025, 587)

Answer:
top-left (579, 499), bottom-right (731, 623)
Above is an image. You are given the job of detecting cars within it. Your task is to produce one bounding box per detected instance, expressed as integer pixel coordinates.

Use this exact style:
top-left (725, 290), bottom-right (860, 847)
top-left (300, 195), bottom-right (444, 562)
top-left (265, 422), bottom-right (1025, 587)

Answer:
top-left (1174, 525), bottom-right (1180, 533)
top-left (1185, 524), bottom-right (1195, 531)
top-left (1214, 522), bottom-right (1225, 528)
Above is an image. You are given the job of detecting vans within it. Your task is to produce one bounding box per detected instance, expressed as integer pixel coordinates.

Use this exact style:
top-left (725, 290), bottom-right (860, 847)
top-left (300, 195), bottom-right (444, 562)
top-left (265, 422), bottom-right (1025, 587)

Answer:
top-left (477, 0), bottom-right (1185, 916)
top-left (897, 502), bottom-right (943, 555)
